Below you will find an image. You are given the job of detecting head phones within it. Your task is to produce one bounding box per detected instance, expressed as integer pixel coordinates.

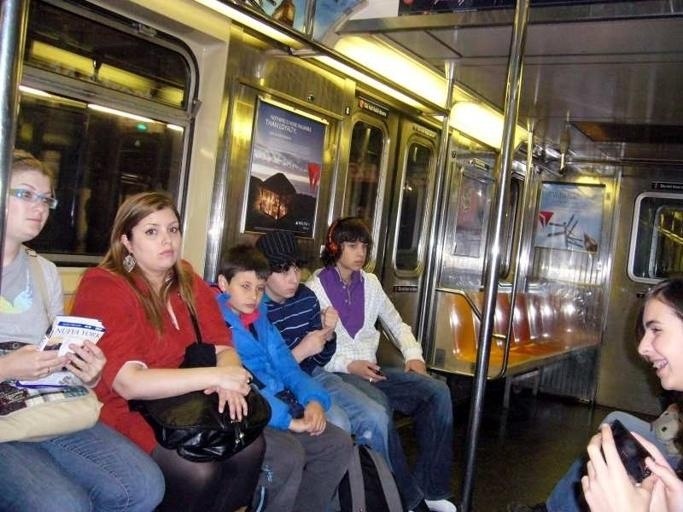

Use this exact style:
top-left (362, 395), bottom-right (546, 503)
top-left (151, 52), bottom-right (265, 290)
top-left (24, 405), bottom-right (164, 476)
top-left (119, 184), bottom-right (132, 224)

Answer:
top-left (325, 216), bottom-right (344, 260)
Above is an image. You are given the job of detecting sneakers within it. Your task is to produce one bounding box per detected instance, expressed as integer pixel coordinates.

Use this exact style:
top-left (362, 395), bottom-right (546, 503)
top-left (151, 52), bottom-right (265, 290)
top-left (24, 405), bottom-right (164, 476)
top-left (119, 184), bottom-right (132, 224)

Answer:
top-left (424, 498), bottom-right (457, 512)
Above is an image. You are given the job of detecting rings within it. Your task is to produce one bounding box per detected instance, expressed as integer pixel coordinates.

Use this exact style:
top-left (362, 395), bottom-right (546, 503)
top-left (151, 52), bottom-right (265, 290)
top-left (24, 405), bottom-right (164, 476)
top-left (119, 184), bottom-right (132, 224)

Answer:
top-left (369, 378), bottom-right (373, 384)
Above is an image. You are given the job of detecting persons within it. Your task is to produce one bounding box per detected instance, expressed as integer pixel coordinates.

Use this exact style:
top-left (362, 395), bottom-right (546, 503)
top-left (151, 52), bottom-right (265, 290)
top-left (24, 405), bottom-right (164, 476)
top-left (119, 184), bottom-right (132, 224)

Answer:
top-left (66, 184), bottom-right (269, 512)
top-left (201, 242), bottom-right (359, 511)
top-left (2, 146), bottom-right (168, 512)
top-left (251, 228), bottom-right (433, 511)
top-left (516, 273), bottom-right (683, 512)
top-left (578, 417), bottom-right (683, 512)
top-left (298, 214), bottom-right (462, 511)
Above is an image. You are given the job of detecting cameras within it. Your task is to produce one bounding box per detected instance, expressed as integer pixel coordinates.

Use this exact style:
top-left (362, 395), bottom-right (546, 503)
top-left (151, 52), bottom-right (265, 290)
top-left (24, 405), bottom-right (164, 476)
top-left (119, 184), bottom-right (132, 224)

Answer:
top-left (601, 418), bottom-right (657, 486)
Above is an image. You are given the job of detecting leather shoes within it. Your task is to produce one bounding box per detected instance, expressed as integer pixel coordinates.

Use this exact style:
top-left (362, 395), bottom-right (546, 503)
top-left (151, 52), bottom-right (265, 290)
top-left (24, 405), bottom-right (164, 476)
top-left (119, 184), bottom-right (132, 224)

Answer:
top-left (506, 501), bottom-right (546, 512)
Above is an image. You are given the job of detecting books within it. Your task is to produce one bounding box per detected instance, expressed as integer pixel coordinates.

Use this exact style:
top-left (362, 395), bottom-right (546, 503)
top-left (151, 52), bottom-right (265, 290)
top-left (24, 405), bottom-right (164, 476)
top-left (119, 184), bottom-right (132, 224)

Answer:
top-left (13, 315), bottom-right (107, 390)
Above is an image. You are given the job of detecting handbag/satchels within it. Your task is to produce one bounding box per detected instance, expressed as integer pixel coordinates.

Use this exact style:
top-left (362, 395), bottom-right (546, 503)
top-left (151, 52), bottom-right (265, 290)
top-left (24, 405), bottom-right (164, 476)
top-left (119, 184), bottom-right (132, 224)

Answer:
top-left (0, 331), bottom-right (104, 443)
top-left (130, 342), bottom-right (272, 464)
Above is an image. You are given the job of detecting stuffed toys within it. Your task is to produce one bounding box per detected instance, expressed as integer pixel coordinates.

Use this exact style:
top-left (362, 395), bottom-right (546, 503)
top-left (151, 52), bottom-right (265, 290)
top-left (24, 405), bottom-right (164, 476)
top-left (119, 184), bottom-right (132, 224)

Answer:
top-left (646, 402), bottom-right (683, 458)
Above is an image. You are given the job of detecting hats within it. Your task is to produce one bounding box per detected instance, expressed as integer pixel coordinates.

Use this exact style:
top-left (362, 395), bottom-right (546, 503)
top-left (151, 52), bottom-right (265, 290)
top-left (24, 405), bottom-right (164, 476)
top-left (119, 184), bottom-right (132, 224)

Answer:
top-left (256, 231), bottom-right (306, 268)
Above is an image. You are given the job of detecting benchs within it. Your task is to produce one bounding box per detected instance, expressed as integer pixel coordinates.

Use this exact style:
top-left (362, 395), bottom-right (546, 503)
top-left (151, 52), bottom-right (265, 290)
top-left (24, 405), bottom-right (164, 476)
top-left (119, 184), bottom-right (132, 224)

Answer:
top-left (432, 290), bottom-right (596, 380)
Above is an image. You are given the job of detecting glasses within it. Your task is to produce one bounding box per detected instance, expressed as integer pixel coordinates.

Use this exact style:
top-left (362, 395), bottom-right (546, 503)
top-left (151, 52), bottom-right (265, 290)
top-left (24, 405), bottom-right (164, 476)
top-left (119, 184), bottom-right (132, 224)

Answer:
top-left (8, 187), bottom-right (58, 210)
top-left (270, 260), bottom-right (302, 273)
